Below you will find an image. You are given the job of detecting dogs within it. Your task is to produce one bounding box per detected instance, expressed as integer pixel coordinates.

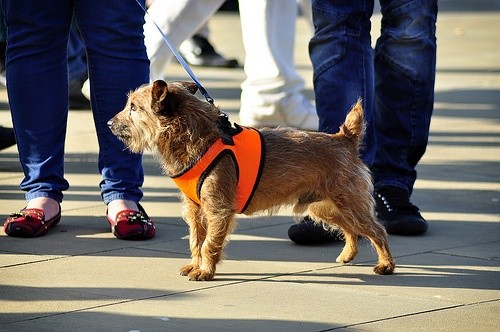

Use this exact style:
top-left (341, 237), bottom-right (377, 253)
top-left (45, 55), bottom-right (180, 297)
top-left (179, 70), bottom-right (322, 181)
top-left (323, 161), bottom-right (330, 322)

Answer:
top-left (106, 79), bottom-right (395, 282)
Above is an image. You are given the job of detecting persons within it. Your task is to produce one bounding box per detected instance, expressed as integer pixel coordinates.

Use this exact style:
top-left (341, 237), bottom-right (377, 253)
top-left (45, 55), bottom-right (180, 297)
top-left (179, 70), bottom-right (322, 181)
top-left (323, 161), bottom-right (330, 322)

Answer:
top-left (0, 0), bottom-right (157, 241)
top-left (81, 0), bottom-right (319, 130)
top-left (287, 0), bottom-right (439, 245)
top-left (178, 20), bottom-right (240, 68)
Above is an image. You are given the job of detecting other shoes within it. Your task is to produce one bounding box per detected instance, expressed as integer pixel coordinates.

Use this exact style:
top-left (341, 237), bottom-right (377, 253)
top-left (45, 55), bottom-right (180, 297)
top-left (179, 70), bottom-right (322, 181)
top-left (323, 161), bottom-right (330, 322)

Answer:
top-left (288, 215), bottom-right (344, 244)
top-left (105, 201), bottom-right (156, 240)
top-left (175, 36), bottom-right (238, 67)
top-left (69, 75), bottom-right (90, 110)
top-left (4, 202), bottom-right (61, 238)
top-left (373, 186), bottom-right (428, 237)
top-left (0, 126), bottom-right (18, 151)
